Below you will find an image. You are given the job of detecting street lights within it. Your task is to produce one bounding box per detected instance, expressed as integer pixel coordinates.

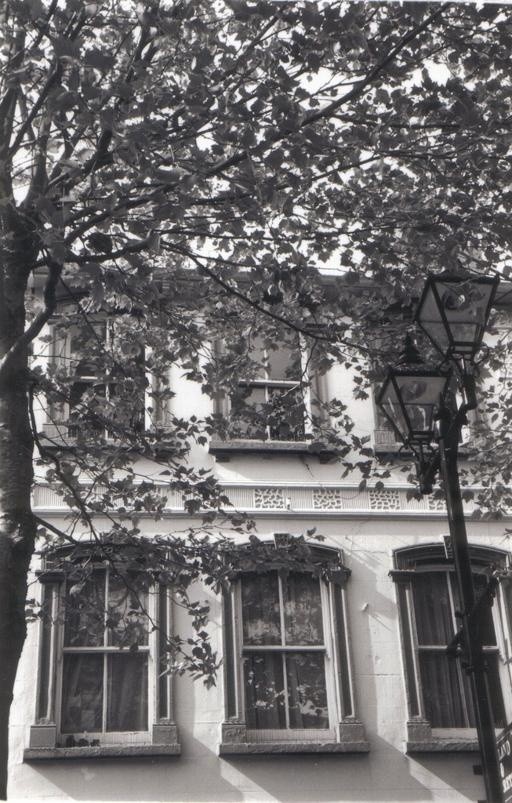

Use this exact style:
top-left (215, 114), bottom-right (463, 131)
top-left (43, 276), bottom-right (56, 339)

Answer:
top-left (370, 253), bottom-right (511, 802)
